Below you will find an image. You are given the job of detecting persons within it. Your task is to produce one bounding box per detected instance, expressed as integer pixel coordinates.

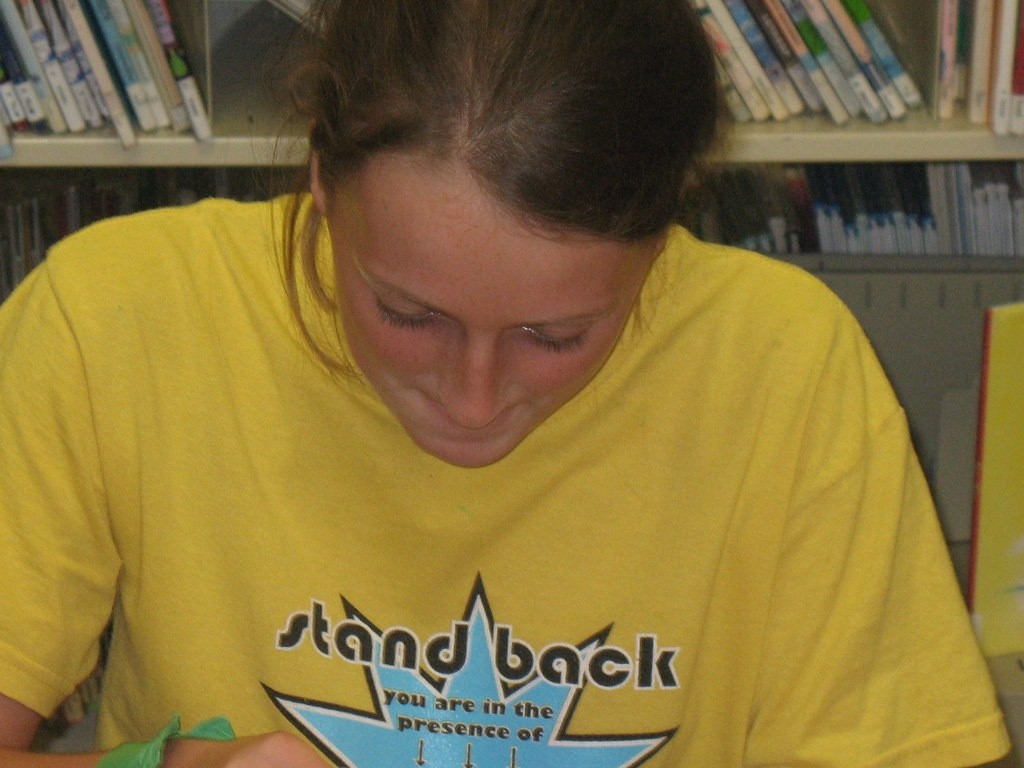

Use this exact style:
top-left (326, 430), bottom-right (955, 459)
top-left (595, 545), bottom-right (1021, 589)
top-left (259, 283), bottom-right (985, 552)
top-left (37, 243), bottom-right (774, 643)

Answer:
top-left (0, 0), bottom-right (1018, 768)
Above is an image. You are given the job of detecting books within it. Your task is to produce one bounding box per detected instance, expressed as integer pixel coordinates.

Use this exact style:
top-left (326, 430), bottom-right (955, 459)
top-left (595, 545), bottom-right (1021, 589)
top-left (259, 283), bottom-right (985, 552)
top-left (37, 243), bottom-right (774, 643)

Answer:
top-left (0, 1), bottom-right (326, 151)
top-left (0, 169), bottom-right (292, 306)
top-left (966, 303), bottom-right (1024, 660)
top-left (688, 0), bottom-right (1024, 137)
top-left (674, 160), bottom-right (1024, 259)
